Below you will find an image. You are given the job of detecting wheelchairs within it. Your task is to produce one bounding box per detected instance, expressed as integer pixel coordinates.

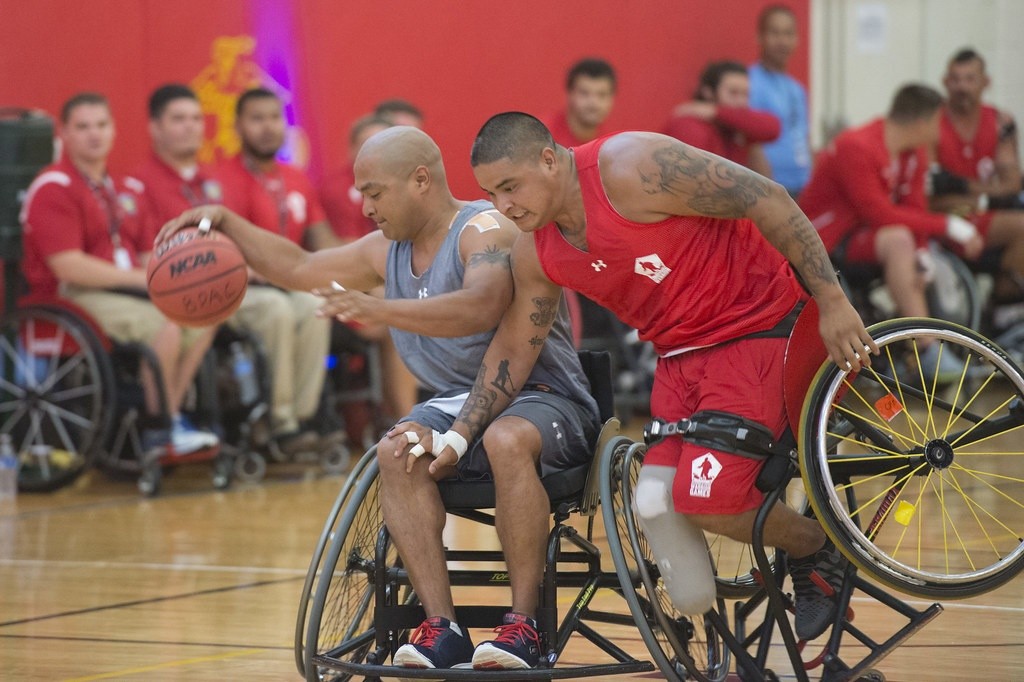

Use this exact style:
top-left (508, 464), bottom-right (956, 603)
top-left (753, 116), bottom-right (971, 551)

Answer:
top-left (295, 351), bottom-right (730, 682)
top-left (0, 278), bottom-right (392, 494)
top-left (624, 243), bottom-right (1024, 682)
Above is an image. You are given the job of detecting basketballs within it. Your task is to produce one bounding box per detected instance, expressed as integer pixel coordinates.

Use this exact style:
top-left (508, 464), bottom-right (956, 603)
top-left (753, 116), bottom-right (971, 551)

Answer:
top-left (146, 227), bottom-right (247, 330)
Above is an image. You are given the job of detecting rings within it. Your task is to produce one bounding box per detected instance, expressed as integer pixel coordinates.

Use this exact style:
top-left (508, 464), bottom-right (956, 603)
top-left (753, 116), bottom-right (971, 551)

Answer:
top-left (404, 432), bottom-right (419, 444)
top-left (409, 444), bottom-right (425, 457)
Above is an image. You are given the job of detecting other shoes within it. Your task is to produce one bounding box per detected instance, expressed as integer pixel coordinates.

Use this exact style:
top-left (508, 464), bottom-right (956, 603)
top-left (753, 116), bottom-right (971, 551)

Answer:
top-left (273, 426), bottom-right (320, 455)
top-left (899, 339), bottom-right (965, 385)
top-left (302, 416), bottom-right (348, 451)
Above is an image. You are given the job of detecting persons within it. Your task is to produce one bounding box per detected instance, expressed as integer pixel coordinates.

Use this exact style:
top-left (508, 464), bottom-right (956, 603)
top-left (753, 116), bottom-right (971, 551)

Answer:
top-left (225, 89), bottom-right (414, 428)
top-left (152, 127), bottom-right (601, 682)
top-left (22, 93), bottom-right (220, 460)
top-left (664, 61), bottom-right (782, 176)
top-left (118, 82), bottom-right (347, 463)
top-left (746, 7), bottom-right (815, 197)
top-left (324, 98), bottom-right (423, 241)
top-left (388, 110), bottom-right (879, 641)
top-left (924, 50), bottom-right (1024, 329)
top-left (799, 82), bottom-right (985, 394)
top-left (554, 54), bottom-right (657, 416)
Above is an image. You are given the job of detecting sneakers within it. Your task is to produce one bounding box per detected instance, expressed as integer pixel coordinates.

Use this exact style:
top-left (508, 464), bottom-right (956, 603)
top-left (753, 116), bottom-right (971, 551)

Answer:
top-left (393, 617), bottom-right (476, 682)
top-left (142, 416), bottom-right (223, 467)
top-left (471, 612), bottom-right (548, 669)
top-left (788, 535), bottom-right (855, 640)
